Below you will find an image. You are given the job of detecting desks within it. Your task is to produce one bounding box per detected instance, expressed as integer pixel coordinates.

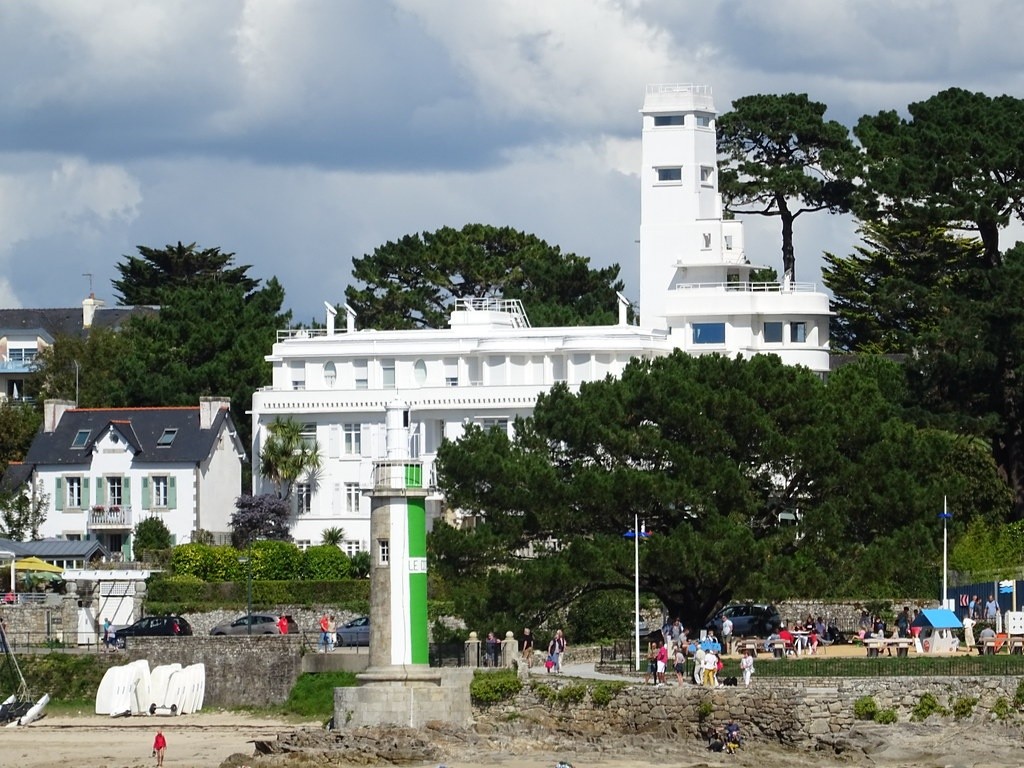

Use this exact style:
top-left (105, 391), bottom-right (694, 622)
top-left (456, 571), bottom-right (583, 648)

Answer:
top-left (791, 631), bottom-right (810, 655)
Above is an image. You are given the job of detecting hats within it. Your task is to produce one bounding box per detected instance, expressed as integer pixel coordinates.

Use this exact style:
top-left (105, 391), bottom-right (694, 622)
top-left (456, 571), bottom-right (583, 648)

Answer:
top-left (279, 613), bottom-right (287, 618)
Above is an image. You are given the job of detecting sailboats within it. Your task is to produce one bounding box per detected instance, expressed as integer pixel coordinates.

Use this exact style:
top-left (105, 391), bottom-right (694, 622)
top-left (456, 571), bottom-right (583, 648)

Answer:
top-left (0, 617), bottom-right (52, 729)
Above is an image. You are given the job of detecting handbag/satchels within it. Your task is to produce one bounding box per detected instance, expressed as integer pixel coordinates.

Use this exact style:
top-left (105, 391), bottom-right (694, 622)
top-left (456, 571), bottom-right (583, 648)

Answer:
top-left (545, 658), bottom-right (554, 668)
top-left (750, 667), bottom-right (755, 674)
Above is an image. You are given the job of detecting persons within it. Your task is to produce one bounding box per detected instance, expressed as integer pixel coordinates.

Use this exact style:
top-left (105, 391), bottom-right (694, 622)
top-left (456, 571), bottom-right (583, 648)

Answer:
top-left (722, 614), bottom-right (733, 655)
top-left (277, 613), bottom-right (288, 634)
top-left (772, 617), bottom-right (826, 658)
top-left (4, 590), bottom-right (14, 604)
top-left (968, 595), bottom-right (982, 618)
top-left (318, 613), bottom-right (335, 653)
top-left (984, 595), bottom-right (1000, 622)
top-left (522, 628), bottom-right (533, 667)
top-left (153, 729), bottom-right (167, 766)
top-left (963, 614), bottom-right (976, 652)
top-left (547, 630), bottom-right (566, 674)
top-left (101, 618), bottom-right (119, 653)
top-left (645, 617), bottom-right (723, 686)
top-left (329, 614), bottom-right (337, 651)
top-left (847, 606), bottom-right (922, 657)
top-left (977, 625), bottom-right (996, 656)
top-left (740, 651), bottom-right (755, 688)
top-left (718, 718), bottom-right (741, 754)
top-left (485, 634), bottom-right (501, 667)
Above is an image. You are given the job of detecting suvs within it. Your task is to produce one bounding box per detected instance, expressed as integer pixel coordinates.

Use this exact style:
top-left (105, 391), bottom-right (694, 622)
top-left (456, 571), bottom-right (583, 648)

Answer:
top-left (702, 602), bottom-right (781, 637)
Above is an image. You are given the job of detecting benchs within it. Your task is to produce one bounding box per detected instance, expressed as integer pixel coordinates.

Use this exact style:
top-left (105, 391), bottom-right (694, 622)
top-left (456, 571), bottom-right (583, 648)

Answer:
top-left (740, 640), bottom-right (798, 657)
top-left (969, 638), bottom-right (1024, 656)
top-left (863, 638), bottom-right (912, 657)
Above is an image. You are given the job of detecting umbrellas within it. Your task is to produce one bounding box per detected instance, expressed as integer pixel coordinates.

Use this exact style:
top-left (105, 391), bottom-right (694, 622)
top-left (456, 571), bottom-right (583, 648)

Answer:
top-left (0, 555), bottom-right (65, 592)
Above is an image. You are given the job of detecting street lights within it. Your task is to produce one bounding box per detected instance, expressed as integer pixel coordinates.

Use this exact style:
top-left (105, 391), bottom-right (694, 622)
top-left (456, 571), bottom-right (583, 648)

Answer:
top-left (937, 494), bottom-right (952, 640)
top-left (621, 513), bottom-right (652, 672)
top-left (238, 528), bottom-right (258, 636)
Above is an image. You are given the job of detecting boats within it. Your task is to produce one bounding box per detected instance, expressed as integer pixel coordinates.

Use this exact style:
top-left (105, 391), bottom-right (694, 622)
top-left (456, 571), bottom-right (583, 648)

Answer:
top-left (93, 657), bottom-right (206, 718)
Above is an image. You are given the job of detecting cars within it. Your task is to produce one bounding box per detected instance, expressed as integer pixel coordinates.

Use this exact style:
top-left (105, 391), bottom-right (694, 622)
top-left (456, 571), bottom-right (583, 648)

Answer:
top-left (113, 616), bottom-right (194, 649)
top-left (334, 615), bottom-right (370, 648)
top-left (209, 611), bottom-right (301, 636)
